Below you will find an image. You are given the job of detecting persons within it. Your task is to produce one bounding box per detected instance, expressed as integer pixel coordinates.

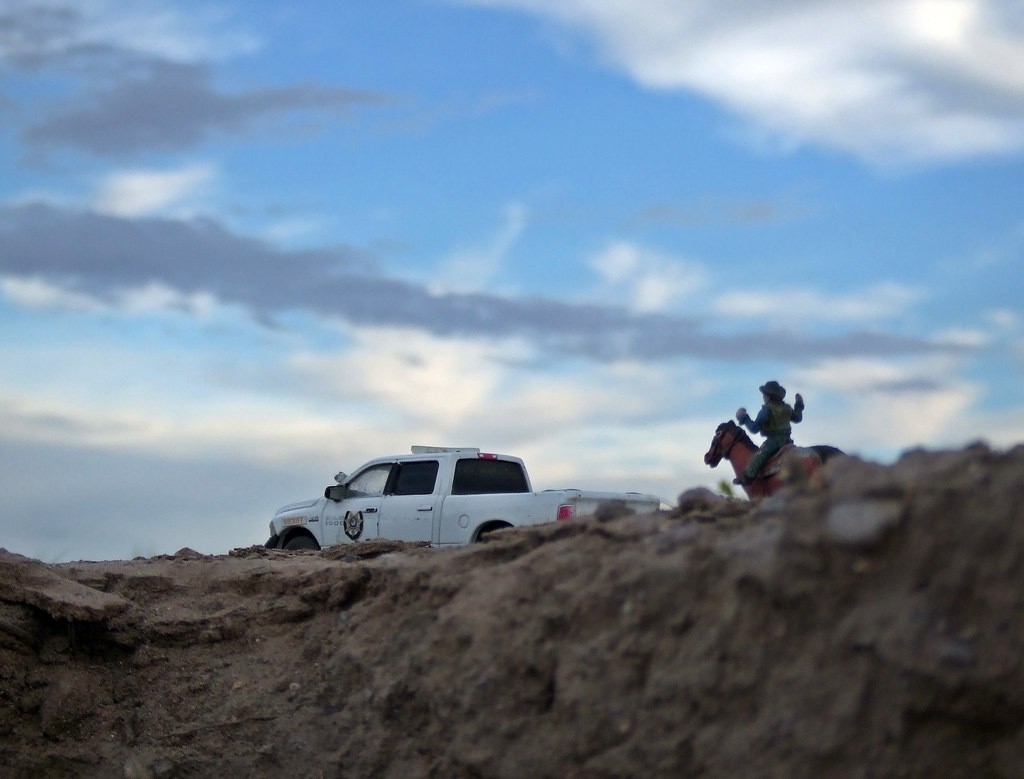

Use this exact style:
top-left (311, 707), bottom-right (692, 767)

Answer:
top-left (733, 381), bottom-right (805, 486)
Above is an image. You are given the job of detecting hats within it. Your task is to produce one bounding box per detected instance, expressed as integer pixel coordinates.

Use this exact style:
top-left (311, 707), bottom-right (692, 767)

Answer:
top-left (759, 381), bottom-right (786, 399)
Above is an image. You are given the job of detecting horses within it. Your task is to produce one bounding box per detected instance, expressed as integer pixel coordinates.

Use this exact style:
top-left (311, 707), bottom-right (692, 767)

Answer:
top-left (704, 420), bottom-right (846, 503)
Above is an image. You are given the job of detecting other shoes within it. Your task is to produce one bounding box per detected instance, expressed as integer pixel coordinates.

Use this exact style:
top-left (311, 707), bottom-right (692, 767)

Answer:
top-left (733, 472), bottom-right (753, 486)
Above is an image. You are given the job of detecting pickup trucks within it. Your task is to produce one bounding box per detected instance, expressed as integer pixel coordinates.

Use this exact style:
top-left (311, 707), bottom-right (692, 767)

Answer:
top-left (265, 446), bottom-right (660, 552)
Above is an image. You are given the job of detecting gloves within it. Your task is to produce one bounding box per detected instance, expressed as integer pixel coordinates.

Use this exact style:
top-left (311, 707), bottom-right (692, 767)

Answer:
top-left (735, 408), bottom-right (747, 420)
top-left (796, 394), bottom-right (803, 405)
